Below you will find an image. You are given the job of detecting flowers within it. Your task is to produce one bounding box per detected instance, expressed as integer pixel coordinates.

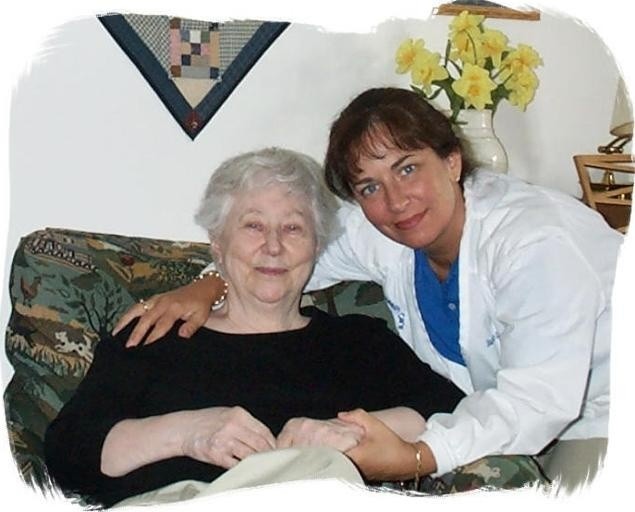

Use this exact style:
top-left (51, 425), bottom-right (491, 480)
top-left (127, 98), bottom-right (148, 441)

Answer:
top-left (396, 10), bottom-right (544, 134)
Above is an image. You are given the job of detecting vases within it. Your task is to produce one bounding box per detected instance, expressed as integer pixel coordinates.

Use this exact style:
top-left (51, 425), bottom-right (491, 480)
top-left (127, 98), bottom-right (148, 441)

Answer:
top-left (440, 108), bottom-right (508, 183)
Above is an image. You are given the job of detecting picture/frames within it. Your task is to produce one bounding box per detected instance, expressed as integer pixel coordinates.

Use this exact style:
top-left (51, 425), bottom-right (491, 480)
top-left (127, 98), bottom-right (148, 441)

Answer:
top-left (433, 2), bottom-right (541, 21)
top-left (98, 16), bottom-right (290, 141)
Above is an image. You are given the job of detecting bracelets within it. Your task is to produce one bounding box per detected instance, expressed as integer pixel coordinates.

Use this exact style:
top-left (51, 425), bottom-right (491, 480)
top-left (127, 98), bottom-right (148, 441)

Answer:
top-left (190, 271), bottom-right (229, 307)
top-left (401, 443), bottom-right (422, 495)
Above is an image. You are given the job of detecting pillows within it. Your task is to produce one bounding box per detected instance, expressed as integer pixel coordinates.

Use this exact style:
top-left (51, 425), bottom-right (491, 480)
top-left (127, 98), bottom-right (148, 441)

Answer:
top-left (4, 229), bottom-right (211, 426)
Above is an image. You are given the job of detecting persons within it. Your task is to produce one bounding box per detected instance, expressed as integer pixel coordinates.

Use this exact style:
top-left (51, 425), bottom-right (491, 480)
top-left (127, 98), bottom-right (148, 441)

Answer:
top-left (112, 86), bottom-right (625, 495)
top-left (41, 145), bottom-right (468, 508)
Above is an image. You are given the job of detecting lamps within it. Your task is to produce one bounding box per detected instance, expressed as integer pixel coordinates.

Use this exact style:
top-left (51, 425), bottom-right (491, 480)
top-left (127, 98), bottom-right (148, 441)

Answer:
top-left (601, 76), bottom-right (634, 192)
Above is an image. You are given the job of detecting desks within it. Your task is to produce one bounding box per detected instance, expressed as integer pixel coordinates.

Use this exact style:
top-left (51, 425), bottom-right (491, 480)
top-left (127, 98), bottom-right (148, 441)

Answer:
top-left (579, 182), bottom-right (632, 236)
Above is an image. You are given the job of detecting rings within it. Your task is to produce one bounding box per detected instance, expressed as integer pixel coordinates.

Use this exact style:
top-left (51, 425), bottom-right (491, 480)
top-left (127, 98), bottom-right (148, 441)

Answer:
top-left (140, 302), bottom-right (149, 312)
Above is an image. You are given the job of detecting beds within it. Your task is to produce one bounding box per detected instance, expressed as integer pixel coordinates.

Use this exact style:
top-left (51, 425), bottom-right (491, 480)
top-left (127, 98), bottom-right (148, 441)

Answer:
top-left (4, 228), bottom-right (556, 511)
